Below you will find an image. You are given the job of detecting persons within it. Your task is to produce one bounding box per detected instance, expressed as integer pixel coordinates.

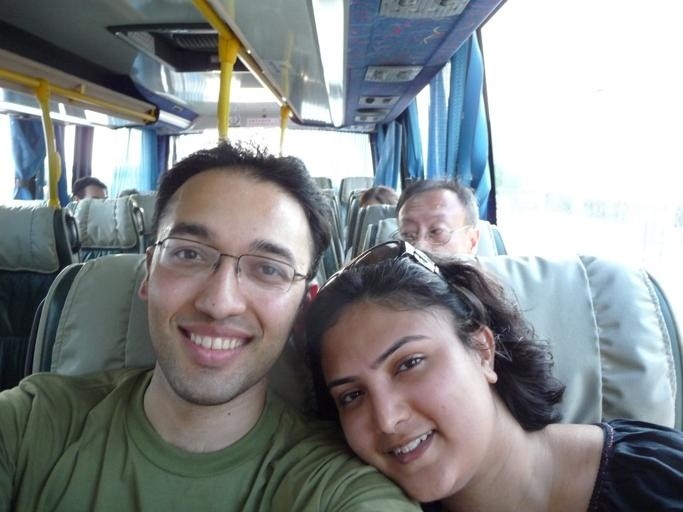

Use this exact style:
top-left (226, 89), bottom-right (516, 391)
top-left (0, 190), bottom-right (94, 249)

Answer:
top-left (68, 176), bottom-right (109, 200)
top-left (299, 237), bottom-right (682, 512)
top-left (358, 186), bottom-right (403, 206)
top-left (0, 142), bottom-right (427, 512)
top-left (394, 177), bottom-right (483, 254)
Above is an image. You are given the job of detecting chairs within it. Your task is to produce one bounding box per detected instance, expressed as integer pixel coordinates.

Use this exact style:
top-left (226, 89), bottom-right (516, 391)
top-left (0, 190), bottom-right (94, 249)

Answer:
top-left (0, 178), bottom-right (683, 430)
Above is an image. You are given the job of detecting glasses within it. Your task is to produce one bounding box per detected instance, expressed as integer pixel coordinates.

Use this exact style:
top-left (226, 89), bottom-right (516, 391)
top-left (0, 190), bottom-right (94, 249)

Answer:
top-left (401, 225), bottom-right (470, 244)
top-left (145, 237), bottom-right (309, 295)
top-left (319, 239), bottom-right (448, 295)
